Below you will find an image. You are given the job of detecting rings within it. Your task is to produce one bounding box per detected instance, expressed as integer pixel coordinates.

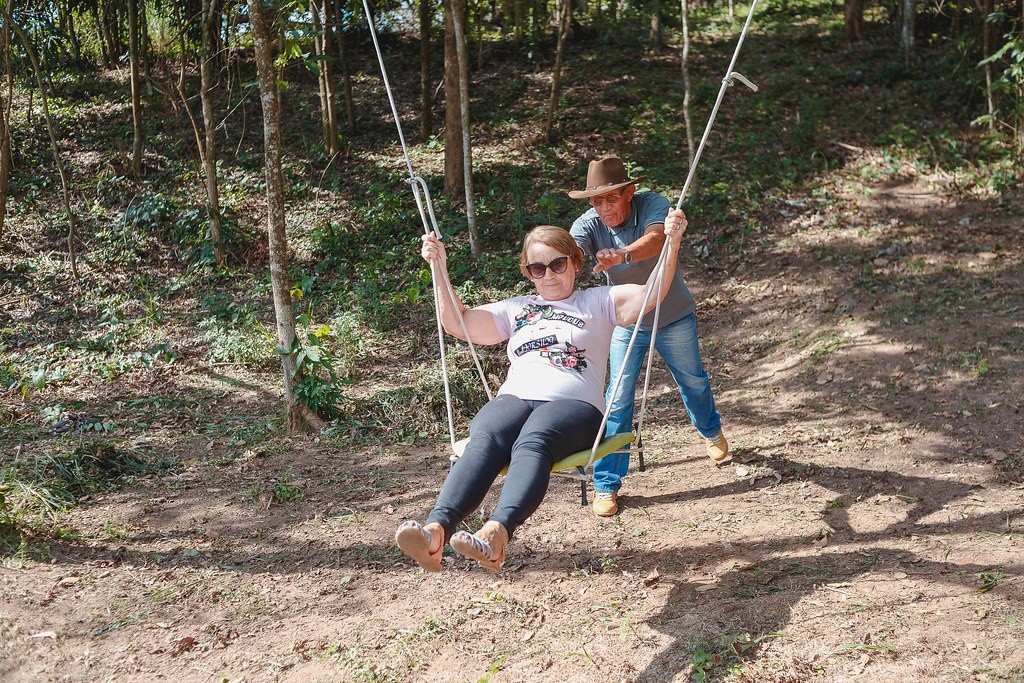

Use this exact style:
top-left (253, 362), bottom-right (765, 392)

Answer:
top-left (676, 223), bottom-right (680, 230)
top-left (679, 218), bottom-right (684, 224)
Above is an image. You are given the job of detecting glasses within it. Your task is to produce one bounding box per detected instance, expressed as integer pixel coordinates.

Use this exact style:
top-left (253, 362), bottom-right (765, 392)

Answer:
top-left (586, 185), bottom-right (628, 207)
top-left (524, 251), bottom-right (575, 279)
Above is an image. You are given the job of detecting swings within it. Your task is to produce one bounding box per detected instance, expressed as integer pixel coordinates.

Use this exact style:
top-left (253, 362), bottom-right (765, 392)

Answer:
top-left (359, 0), bottom-right (759, 474)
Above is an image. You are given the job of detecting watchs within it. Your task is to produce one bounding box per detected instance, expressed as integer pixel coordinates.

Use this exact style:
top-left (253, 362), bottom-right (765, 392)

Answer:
top-left (618, 249), bottom-right (632, 264)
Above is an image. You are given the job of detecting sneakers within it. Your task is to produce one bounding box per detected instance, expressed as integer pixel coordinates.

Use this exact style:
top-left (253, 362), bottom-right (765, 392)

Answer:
top-left (702, 426), bottom-right (729, 462)
top-left (593, 486), bottom-right (619, 517)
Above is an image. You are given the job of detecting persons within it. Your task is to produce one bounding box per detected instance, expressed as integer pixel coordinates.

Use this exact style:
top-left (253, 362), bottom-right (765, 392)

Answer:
top-left (394, 206), bottom-right (690, 574)
top-left (566, 157), bottom-right (730, 519)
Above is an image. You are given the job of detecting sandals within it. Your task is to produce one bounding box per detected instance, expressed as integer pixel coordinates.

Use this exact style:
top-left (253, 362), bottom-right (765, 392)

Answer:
top-left (394, 520), bottom-right (444, 572)
top-left (448, 529), bottom-right (508, 573)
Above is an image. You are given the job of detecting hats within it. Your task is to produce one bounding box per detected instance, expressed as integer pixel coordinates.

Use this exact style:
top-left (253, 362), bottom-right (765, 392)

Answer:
top-left (569, 155), bottom-right (651, 199)
